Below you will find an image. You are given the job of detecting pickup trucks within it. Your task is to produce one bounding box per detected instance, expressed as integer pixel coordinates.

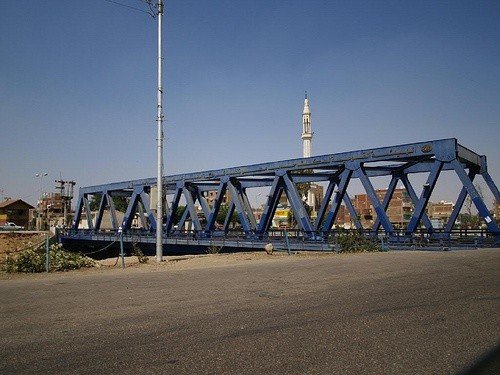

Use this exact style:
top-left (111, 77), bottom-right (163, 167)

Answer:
top-left (0, 222), bottom-right (25, 231)
